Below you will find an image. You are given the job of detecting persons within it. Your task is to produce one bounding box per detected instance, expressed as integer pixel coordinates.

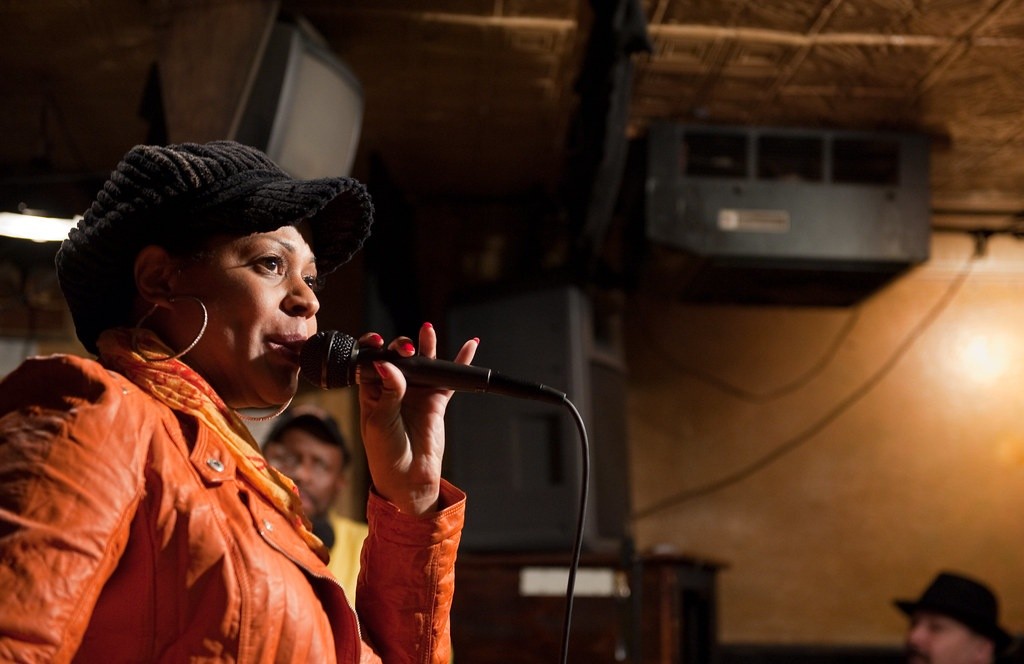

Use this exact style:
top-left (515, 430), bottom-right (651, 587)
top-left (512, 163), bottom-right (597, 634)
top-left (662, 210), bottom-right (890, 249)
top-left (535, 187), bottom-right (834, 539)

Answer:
top-left (1, 142), bottom-right (484, 664)
top-left (890, 569), bottom-right (1018, 664)
top-left (259, 411), bottom-right (372, 643)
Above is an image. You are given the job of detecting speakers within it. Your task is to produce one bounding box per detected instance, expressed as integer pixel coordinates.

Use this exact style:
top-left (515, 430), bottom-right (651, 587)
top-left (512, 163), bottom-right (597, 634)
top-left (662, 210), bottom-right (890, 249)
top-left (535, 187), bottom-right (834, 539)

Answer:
top-left (406, 281), bottom-right (633, 558)
top-left (621, 120), bottom-right (931, 308)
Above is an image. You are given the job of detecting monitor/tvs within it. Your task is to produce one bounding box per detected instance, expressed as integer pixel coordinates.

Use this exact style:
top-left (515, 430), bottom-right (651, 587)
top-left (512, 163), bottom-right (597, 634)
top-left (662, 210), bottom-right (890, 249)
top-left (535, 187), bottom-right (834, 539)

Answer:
top-left (230, 24), bottom-right (363, 183)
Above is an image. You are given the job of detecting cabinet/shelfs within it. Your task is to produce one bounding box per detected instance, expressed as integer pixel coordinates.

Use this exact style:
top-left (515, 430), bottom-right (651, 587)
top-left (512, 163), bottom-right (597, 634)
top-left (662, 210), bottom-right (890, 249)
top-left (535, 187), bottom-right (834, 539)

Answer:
top-left (447, 554), bottom-right (725, 664)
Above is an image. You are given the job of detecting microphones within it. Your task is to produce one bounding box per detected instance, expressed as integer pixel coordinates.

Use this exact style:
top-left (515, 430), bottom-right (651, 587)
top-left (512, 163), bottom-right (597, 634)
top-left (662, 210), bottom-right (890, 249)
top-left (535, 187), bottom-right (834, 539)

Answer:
top-left (301, 331), bottom-right (567, 410)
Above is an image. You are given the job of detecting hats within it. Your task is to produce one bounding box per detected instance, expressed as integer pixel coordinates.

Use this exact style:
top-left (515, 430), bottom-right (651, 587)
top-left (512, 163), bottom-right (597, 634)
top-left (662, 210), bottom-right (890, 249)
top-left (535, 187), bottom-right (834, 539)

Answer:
top-left (893, 571), bottom-right (1013, 654)
top-left (54, 139), bottom-right (375, 352)
